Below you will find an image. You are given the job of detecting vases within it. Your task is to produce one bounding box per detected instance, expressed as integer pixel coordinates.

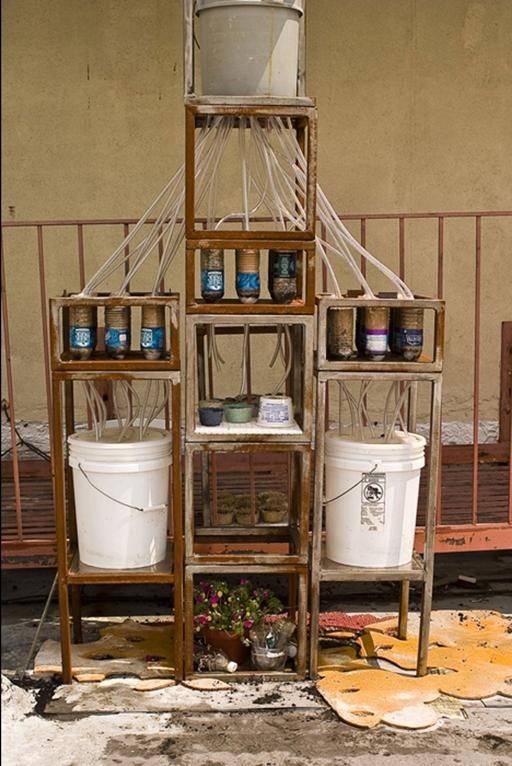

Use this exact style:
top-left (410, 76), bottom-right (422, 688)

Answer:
top-left (201, 628), bottom-right (246, 667)
top-left (251, 643), bottom-right (287, 673)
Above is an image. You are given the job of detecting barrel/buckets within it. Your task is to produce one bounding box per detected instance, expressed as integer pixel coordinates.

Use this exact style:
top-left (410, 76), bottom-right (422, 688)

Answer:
top-left (66, 426), bottom-right (173, 571)
top-left (321, 430), bottom-right (426, 570)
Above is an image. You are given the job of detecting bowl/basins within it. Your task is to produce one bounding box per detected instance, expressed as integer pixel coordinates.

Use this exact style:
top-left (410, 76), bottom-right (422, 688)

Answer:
top-left (199, 395), bottom-right (260, 425)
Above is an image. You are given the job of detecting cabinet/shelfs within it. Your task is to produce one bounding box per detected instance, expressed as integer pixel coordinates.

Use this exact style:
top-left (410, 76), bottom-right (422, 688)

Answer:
top-left (315, 289), bottom-right (442, 679)
top-left (47, 286), bottom-right (185, 686)
top-left (178, 98), bottom-right (317, 679)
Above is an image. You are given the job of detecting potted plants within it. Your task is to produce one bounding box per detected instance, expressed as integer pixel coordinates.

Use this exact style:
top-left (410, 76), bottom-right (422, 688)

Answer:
top-left (210, 484), bottom-right (288, 528)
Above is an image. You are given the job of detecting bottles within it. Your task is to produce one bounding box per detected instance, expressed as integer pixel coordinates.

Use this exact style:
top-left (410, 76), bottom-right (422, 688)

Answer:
top-left (327, 307), bottom-right (423, 363)
top-left (209, 651), bottom-right (239, 674)
top-left (199, 247), bottom-right (297, 303)
top-left (68, 304), bottom-right (166, 361)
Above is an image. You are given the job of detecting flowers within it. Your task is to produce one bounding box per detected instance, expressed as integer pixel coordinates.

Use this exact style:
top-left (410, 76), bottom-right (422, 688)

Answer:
top-left (194, 577), bottom-right (281, 635)
top-left (248, 612), bottom-right (297, 643)
